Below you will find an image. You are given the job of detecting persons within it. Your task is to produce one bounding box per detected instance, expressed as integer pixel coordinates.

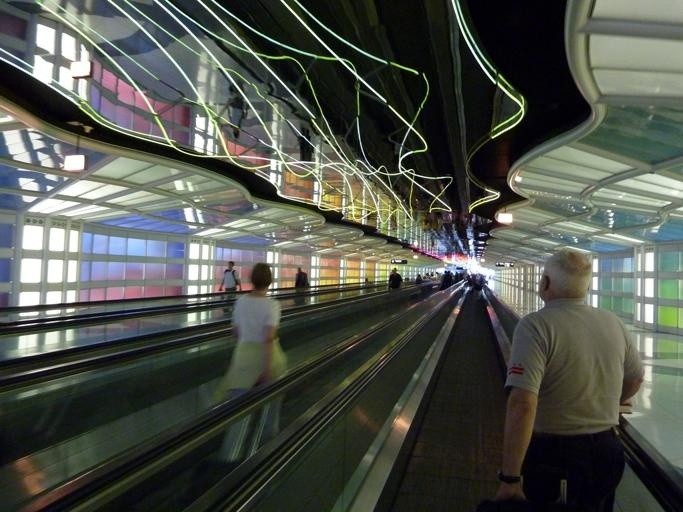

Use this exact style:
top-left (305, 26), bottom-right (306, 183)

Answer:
top-left (364, 278), bottom-right (369, 288)
top-left (489, 245), bottom-right (645, 511)
top-left (218, 260), bottom-right (242, 313)
top-left (212, 263), bottom-right (289, 482)
top-left (295, 267), bottom-right (308, 305)
top-left (388, 268), bottom-right (489, 291)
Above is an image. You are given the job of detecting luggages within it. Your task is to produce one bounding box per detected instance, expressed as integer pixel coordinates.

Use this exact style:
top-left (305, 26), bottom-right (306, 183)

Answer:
top-left (220, 385), bottom-right (271, 462)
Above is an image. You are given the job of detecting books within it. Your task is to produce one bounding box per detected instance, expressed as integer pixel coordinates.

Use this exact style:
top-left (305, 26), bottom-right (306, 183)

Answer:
top-left (619, 402), bottom-right (633, 415)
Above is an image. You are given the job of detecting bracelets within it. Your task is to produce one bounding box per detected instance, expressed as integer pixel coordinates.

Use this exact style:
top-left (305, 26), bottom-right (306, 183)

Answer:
top-left (496, 469), bottom-right (521, 484)
